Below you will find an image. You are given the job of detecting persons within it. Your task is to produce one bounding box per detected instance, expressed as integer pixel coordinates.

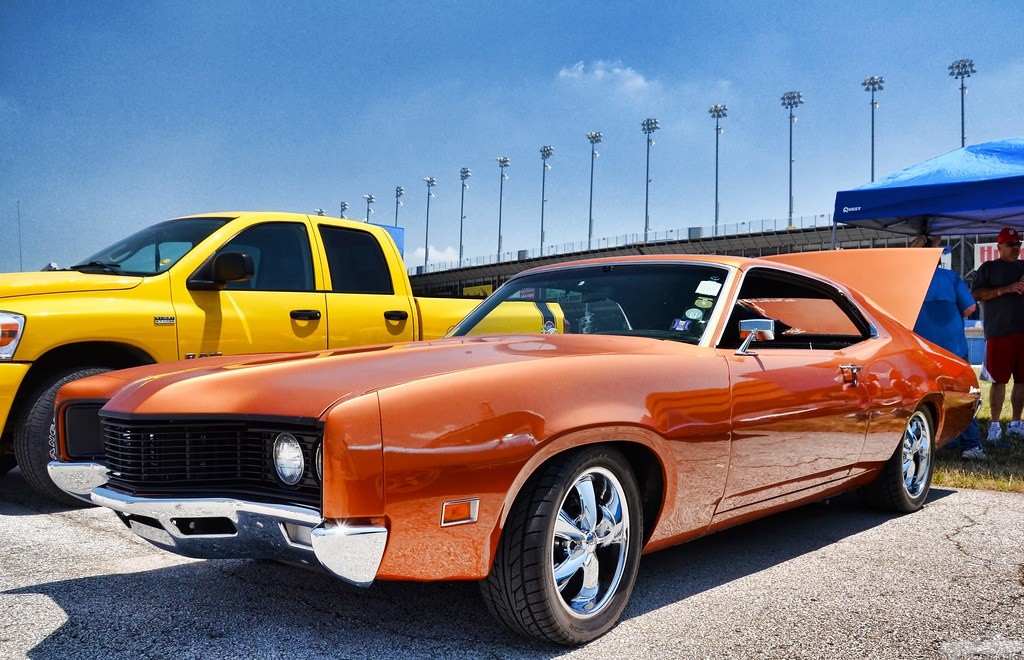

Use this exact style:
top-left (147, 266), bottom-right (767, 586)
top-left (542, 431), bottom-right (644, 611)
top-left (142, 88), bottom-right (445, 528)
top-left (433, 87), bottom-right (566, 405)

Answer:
top-left (914, 258), bottom-right (987, 459)
top-left (970, 227), bottom-right (1024, 442)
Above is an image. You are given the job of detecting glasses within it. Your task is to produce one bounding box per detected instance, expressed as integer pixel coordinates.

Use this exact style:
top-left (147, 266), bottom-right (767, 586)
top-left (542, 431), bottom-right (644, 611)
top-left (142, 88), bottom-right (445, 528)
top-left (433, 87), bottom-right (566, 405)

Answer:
top-left (1000, 242), bottom-right (1022, 247)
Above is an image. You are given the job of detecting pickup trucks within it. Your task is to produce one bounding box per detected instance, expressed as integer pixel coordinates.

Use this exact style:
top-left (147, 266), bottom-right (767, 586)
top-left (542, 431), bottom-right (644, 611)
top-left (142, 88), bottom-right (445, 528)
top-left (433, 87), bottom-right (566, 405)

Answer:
top-left (0, 206), bottom-right (569, 514)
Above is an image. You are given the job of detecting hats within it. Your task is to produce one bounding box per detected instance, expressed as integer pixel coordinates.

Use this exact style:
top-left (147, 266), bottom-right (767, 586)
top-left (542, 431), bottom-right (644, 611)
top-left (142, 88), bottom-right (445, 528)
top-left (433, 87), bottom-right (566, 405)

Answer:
top-left (998, 228), bottom-right (1024, 243)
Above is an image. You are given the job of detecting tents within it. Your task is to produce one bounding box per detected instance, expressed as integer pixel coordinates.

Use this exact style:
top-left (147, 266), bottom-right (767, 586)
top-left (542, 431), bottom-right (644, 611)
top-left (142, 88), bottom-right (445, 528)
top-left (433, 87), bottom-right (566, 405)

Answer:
top-left (830, 138), bottom-right (1024, 282)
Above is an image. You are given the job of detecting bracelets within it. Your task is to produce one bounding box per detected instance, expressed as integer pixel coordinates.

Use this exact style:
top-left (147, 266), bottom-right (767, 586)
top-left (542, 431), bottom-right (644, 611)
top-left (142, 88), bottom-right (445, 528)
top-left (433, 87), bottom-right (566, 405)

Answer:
top-left (997, 289), bottom-right (1000, 296)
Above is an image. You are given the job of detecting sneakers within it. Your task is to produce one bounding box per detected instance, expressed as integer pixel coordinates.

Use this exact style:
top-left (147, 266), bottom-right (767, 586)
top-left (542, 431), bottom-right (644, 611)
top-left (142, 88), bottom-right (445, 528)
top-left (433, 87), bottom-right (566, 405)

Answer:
top-left (962, 447), bottom-right (986, 460)
top-left (1006, 422), bottom-right (1024, 437)
top-left (986, 424), bottom-right (1002, 442)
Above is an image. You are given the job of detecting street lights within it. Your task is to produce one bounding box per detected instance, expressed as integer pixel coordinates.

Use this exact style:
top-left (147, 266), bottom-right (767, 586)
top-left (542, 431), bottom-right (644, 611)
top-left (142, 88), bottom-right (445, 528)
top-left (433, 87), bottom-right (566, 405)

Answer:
top-left (393, 186), bottom-right (404, 227)
top-left (583, 131), bottom-right (604, 250)
top-left (640, 117), bottom-right (661, 243)
top-left (458, 166), bottom-right (471, 268)
top-left (948, 58), bottom-right (977, 147)
top-left (495, 156), bottom-right (511, 263)
top-left (363, 194), bottom-right (375, 222)
top-left (781, 90), bottom-right (805, 230)
top-left (708, 102), bottom-right (729, 238)
top-left (860, 75), bottom-right (885, 183)
top-left (539, 145), bottom-right (554, 257)
top-left (422, 176), bottom-right (437, 274)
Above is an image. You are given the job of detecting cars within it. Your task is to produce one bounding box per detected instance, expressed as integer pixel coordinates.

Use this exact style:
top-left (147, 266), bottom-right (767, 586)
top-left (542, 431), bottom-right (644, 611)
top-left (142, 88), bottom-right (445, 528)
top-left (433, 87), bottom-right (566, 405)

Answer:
top-left (45, 248), bottom-right (984, 651)
